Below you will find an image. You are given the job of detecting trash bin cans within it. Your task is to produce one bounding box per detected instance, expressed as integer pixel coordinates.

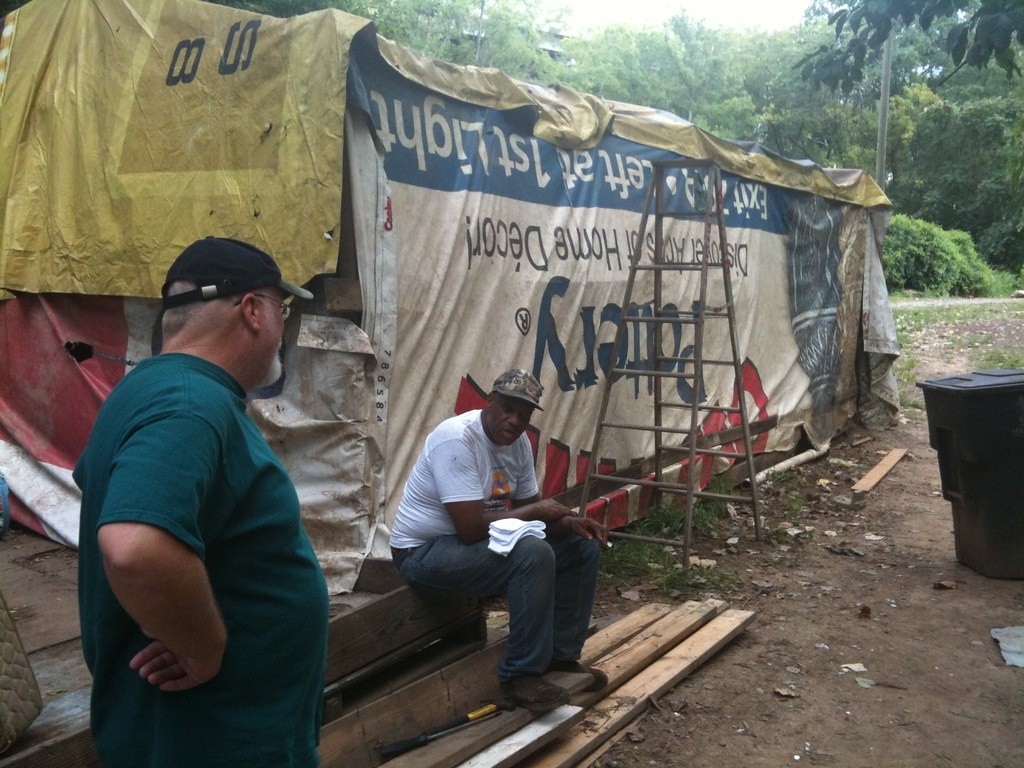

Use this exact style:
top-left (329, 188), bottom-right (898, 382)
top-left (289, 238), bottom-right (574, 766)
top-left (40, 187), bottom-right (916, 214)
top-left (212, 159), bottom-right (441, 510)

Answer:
top-left (915, 370), bottom-right (1024, 580)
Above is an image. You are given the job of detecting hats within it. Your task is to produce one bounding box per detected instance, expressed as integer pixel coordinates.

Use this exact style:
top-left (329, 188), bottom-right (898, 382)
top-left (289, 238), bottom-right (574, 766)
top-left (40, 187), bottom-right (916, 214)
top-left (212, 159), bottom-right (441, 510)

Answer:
top-left (161, 235), bottom-right (314, 310)
top-left (492, 368), bottom-right (545, 413)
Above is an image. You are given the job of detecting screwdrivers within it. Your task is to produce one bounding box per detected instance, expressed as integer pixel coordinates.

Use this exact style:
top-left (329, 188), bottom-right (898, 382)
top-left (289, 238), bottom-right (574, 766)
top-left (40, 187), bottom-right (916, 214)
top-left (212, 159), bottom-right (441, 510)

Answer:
top-left (381, 711), bottom-right (502, 758)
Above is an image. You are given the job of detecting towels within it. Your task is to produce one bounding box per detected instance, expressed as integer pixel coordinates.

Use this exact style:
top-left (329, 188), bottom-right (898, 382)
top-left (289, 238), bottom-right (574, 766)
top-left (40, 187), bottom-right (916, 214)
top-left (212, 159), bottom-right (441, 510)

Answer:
top-left (487, 517), bottom-right (547, 557)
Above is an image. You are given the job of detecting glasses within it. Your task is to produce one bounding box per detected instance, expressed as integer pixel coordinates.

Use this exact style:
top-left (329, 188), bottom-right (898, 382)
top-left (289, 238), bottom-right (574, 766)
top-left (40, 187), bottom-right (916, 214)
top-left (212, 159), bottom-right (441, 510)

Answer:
top-left (233, 293), bottom-right (291, 322)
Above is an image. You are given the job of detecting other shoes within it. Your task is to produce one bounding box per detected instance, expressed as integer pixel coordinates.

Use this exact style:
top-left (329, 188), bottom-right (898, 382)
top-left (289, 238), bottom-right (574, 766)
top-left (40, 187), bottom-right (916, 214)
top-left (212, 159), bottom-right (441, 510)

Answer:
top-left (501, 678), bottom-right (572, 714)
top-left (550, 657), bottom-right (607, 691)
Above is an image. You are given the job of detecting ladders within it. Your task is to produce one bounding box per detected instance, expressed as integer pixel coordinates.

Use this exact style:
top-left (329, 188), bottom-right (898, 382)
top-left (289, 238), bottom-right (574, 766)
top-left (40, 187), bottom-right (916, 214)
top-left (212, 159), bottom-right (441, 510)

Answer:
top-left (579, 158), bottom-right (762, 569)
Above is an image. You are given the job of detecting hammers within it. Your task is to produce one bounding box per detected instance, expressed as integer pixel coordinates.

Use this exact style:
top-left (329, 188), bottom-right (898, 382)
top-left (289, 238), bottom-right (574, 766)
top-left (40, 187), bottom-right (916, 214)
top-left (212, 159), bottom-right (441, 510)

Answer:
top-left (423, 697), bottom-right (516, 737)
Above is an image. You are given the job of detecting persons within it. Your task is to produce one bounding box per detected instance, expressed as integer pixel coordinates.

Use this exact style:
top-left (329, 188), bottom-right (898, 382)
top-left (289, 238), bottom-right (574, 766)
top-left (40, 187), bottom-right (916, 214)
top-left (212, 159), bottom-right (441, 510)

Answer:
top-left (72, 235), bottom-right (331, 768)
top-left (390, 368), bottom-right (609, 711)
